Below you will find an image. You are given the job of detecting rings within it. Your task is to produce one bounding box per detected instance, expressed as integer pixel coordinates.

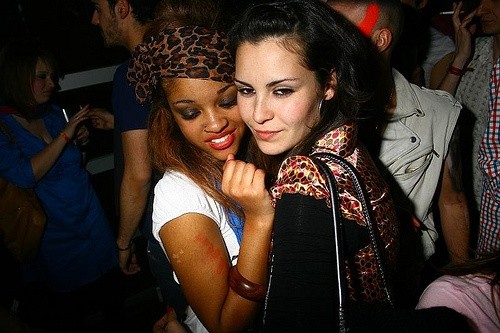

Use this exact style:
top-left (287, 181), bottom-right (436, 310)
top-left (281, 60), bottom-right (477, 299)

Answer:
top-left (75, 116), bottom-right (79, 120)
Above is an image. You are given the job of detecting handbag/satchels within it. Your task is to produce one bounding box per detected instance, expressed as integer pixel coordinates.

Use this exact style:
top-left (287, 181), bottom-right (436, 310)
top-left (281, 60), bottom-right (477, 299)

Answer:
top-left (0, 120), bottom-right (46, 260)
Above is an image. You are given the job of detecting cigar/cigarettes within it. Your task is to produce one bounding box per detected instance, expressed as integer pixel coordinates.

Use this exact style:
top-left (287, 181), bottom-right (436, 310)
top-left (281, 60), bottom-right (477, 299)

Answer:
top-left (80, 104), bottom-right (83, 111)
top-left (440, 11), bottom-right (464, 14)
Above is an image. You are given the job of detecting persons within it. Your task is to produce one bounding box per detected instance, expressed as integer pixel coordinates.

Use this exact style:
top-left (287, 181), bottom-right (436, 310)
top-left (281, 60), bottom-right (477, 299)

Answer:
top-left (91, 0), bottom-right (189, 324)
top-left (325, 0), bottom-right (500, 286)
top-left (127, 0), bottom-right (421, 333)
top-left (414, 247), bottom-right (500, 333)
top-left (0, 43), bottom-right (123, 281)
top-left (153, 0), bottom-right (400, 333)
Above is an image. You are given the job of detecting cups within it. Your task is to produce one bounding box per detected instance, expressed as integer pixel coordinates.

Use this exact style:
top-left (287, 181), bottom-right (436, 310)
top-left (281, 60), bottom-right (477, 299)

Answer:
top-left (58, 108), bottom-right (74, 125)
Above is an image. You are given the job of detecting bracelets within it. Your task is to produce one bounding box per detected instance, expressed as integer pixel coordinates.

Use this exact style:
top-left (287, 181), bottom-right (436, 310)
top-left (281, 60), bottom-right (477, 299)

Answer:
top-left (228, 265), bottom-right (267, 303)
top-left (448, 64), bottom-right (464, 76)
top-left (60, 132), bottom-right (69, 142)
top-left (120, 242), bottom-right (133, 250)
